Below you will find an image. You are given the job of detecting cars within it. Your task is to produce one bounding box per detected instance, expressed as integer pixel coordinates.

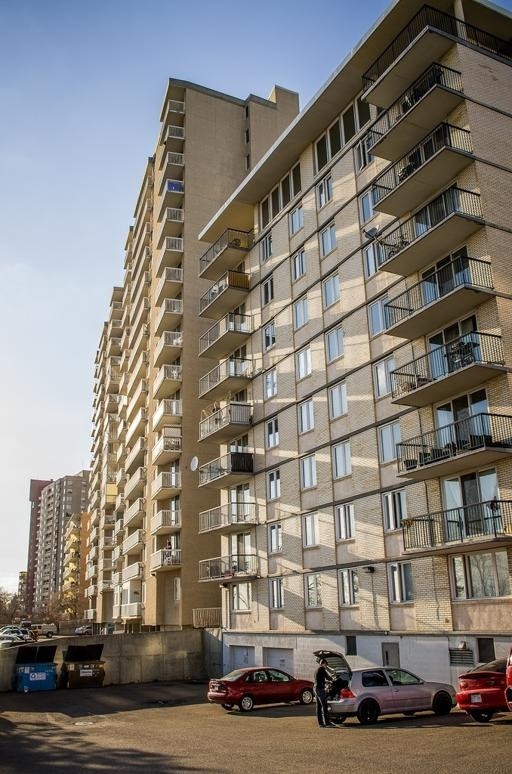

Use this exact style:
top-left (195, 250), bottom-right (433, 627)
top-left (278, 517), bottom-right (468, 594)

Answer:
top-left (75, 624), bottom-right (92, 635)
top-left (207, 666), bottom-right (314, 712)
top-left (312, 649), bottom-right (511, 725)
top-left (0, 623), bottom-right (57, 644)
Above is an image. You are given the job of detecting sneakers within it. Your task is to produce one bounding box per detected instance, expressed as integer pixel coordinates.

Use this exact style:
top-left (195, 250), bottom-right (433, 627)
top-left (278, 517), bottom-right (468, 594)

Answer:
top-left (319, 721), bottom-right (341, 729)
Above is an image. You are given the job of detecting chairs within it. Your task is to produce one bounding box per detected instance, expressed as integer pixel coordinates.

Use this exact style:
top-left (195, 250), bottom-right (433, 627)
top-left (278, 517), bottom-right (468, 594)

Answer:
top-left (404, 434), bottom-right (491, 470)
top-left (255, 673), bottom-right (261, 681)
top-left (260, 674), bottom-right (267, 682)
top-left (389, 675), bottom-right (402, 685)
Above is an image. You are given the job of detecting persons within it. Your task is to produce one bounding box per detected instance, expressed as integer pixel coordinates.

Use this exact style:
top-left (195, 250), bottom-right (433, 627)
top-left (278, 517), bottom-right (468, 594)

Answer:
top-left (311, 656), bottom-right (339, 728)
